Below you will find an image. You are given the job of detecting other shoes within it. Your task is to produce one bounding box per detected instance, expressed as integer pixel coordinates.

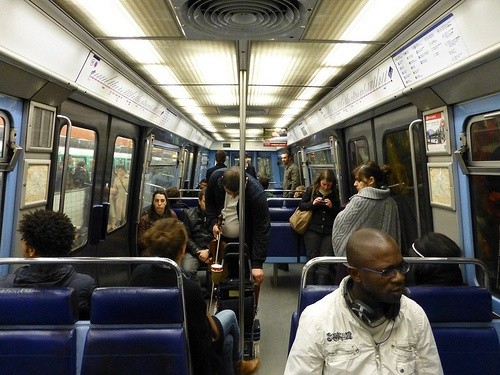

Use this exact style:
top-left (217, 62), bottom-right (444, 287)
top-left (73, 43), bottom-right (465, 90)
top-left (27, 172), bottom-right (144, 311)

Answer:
top-left (234, 358), bottom-right (259, 375)
top-left (277, 263), bottom-right (289, 272)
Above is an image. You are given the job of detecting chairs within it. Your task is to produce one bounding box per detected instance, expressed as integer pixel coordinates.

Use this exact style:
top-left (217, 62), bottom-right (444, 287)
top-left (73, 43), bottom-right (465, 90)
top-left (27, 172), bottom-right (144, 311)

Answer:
top-left (0, 188), bottom-right (500, 375)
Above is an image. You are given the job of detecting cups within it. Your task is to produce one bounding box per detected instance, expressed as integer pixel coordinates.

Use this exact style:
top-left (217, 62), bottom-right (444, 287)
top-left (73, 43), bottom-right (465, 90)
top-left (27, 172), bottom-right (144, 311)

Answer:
top-left (211, 264), bottom-right (222, 271)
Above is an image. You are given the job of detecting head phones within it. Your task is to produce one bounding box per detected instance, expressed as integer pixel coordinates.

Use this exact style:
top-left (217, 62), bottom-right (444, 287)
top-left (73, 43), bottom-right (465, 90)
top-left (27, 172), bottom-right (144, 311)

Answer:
top-left (344, 283), bottom-right (400, 325)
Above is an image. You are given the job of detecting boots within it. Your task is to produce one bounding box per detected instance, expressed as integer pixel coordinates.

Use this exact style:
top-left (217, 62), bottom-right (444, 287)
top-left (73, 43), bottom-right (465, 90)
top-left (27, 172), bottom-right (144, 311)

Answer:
top-left (316, 270), bottom-right (331, 285)
top-left (306, 263), bottom-right (320, 285)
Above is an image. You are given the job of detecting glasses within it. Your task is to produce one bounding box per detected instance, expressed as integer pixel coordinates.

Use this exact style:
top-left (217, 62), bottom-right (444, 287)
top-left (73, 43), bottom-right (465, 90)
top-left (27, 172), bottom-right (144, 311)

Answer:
top-left (357, 262), bottom-right (410, 278)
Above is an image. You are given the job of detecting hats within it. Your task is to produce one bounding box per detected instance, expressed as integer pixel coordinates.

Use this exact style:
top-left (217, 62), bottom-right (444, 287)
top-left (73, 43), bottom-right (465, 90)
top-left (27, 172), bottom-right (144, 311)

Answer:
top-left (412, 232), bottom-right (462, 258)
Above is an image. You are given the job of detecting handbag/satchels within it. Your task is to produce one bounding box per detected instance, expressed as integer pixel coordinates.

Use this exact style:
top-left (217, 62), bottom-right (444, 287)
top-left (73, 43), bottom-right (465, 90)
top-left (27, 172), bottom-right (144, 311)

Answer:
top-left (289, 185), bottom-right (315, 234)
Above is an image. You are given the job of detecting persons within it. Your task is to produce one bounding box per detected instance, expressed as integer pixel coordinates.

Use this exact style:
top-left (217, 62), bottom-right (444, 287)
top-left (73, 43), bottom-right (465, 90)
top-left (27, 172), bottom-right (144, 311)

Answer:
top-left (184, 189), bottom-right (214, 280)
top-left (289, 170), bottom-right (341, 285)
top-left (196, 150), bottom-right (269, 190)
top-left (0, 208), bottom-right (102, 320)
top-left (58, 153), bottom-right (94, 190)
top-left (206, 166), bottom-right (271, 286)
top-left (408, 232), bottom-right (460, 287)
top-left (293, 186), bottom-right (307, 198)
top-left (108, 164), bottom-right (129, 229)
top-left (332, 160), bottom-right (402, 284)
top-left (129, 219), bottom-right (260, 375)
top-left (280, 150), bottom-right (307, 207)
top-left (284, 228), bottom-right (444, 375)
top-left (138, 190), bottom-right (180, 234)
